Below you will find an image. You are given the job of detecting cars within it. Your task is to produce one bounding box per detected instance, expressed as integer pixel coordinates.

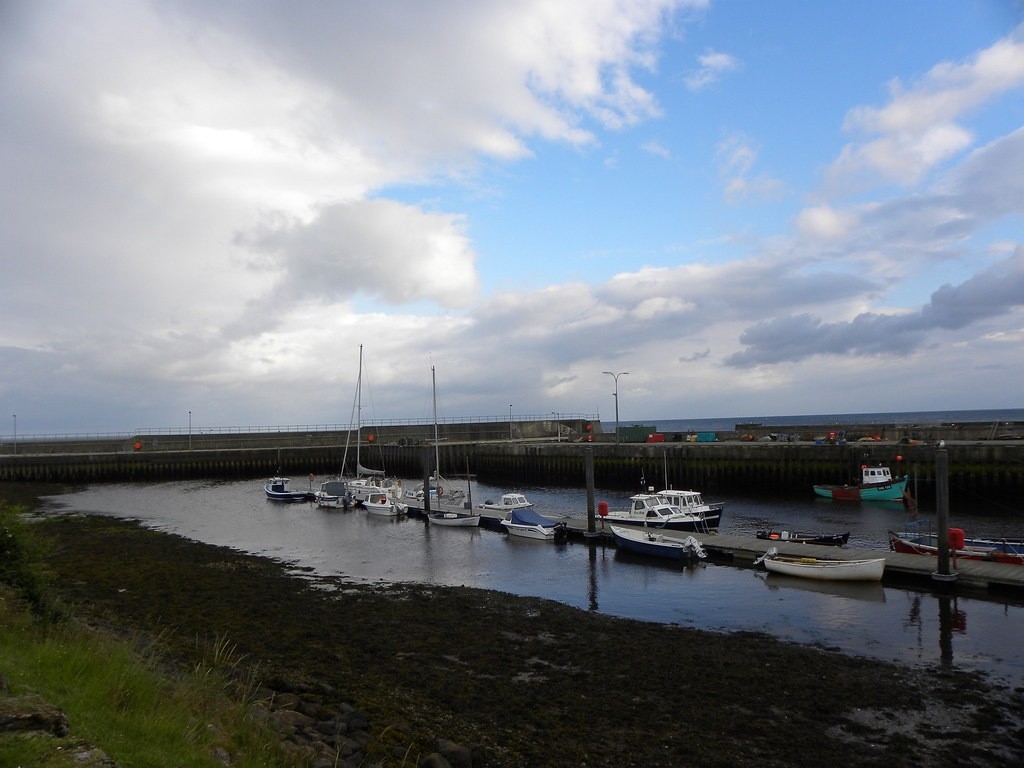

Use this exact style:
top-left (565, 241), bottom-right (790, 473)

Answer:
top-left (398, 436), bottom-right (422, 445)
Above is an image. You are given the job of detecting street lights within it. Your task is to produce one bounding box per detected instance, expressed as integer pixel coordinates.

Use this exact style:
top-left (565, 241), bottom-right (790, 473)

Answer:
top-left (603, 371), bottom-right (629, 443)
top-left (13, 414), bottom-right (16, 454)
top-left (189, 412), bottom-right (192, 449)
top-left (510, 404), bottom-right (512, 440)
top-left (552, 412), bottom-right (561, 442)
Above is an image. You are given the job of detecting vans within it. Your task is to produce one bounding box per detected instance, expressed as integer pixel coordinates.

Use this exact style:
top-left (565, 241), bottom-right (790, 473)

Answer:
top-left (647, 434), bottom-right (665, 443)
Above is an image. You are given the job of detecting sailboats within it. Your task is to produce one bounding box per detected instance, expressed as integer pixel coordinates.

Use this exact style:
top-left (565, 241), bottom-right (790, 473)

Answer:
top-left (340, 343), bottom-right (403, 499)
top-left (427, 457), bottom-right (481, 526)
top-left (813, 445), bottom-right (910, 502)
top-left (888, 464), bottom-right (1024, 565)
top-left (264, 442), bottom-right (308, 500)
top-left (405, 351), bottom-right (464, 506)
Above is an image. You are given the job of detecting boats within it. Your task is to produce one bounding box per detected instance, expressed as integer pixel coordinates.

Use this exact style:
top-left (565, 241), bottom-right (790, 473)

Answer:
top-left (756, 529), bottom-right (850, 548)
top-left (499, 508), bottom-right (566, 540)
top-left (479, 492), bottom-right (534, 512)
top-left (595, 452), bottom-right (724, 534)
top-left (753, 547), bottom-right (886, 582)
top-left (610, 525), bottom-right (708, 567)
top-left (313, 481), bottom-right (356, 508)
top-left (362, 492), bottom-right (408, 515)
